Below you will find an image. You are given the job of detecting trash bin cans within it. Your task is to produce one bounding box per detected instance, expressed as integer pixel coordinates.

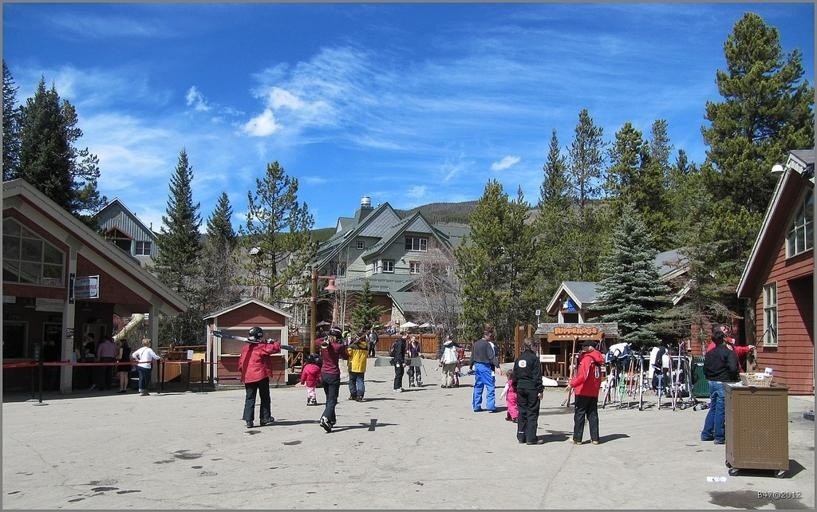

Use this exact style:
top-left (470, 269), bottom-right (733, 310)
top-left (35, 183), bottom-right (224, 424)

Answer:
top-left (692, 356), bottom-right (712, 398)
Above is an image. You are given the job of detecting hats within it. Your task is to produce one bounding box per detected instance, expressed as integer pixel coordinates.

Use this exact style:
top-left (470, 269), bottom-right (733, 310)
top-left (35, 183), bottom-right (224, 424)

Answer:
top-left (579, 340), bottom-right (596, 348)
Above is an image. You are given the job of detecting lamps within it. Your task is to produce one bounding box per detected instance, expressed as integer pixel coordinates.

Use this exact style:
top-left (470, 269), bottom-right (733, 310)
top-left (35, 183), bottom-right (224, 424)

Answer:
top-left (769, 160), bottom-right (788, 176)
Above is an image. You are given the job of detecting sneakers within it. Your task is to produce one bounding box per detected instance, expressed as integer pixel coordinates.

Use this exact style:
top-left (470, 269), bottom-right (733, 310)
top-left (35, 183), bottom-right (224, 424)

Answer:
top-left (260, 416), bottom-right (274, 427)
top-left (116, 389), bottom-right (150, 397)
top-left (348, 393), bottom-right (365, 402)
top-left (526, 439), bottom-right (545, 445)
top-left (246, 420), bottom-right (254, 428)
top-left (320, 417), bottom-right (336, 434)
top-left (518, 439), bottom-right (526, 444)
top-left (590, 439), bottom-right (599, 444)
top-left (393, 387), bottom-right (404, 393)
top-left (307, 397), bottom-right (318, 406)
top-left (569, 437), bottom-right (583, 445)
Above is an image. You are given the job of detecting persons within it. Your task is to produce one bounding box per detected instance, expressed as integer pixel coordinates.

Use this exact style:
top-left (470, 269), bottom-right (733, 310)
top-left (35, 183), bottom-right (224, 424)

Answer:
top-left (300, 352), bottom-right (321, 406)
top-left (389, 331), bottom-right (408, 394)
top-left (365, 326), bottom-right (379, 359)
top-left (561, 340), bottom-right (605, 446)
top-left (698, 331), bottom-right (740, 446)
top-left (345, 331), bottom-right (369, 403)
top-left (404, 335), bottom-right (424, 386)
top-left (466, 329), bottom-right (500, 415)
top-left (510, 336), bottom-right (545, 445)
top-left (317, 326), bottom-right (347, 433)
top-left (32, 328), bottom-right (160, 397)
top-left (238, 326), bottom-right (282, 430)
top-left (453, 343), bottom-right (465, 390)
top-left (435, 339), bottom-right (458, 390)
top-left (704, 323), bottom-right (756, 373)
top-left (497, 367), bottom-right (520, 424)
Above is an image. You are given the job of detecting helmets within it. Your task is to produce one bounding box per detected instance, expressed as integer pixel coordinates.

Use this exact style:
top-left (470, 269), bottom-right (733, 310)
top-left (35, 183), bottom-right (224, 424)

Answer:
top-left (330, 328), bottom-right (343, 340)
top-left (248, 327), bottom-right (264, 339)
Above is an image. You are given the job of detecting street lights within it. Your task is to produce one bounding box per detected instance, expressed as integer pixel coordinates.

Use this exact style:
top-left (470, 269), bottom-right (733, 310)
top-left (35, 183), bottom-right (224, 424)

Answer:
top-left (249, 246), bottom-right (262, 298)
top-left (304, 265), bottom-right (338, 362)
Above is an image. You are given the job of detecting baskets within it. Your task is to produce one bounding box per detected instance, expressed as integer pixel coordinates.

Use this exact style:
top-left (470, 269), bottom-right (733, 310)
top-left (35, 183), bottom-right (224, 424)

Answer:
top-left (738, 371), bottom-right (773, 388)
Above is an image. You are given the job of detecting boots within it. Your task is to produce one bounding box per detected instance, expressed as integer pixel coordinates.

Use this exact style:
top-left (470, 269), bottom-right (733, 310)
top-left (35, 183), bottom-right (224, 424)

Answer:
top-left (409, 375), bottom-right (415, 388)
top-left (416, 373), bottom-right (423, 387)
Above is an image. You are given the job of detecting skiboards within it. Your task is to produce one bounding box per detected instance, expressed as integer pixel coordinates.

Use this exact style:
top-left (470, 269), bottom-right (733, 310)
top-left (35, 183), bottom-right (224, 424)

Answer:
top-left (213, 329), bottom-right (297, 352)
top-left (561, 342), bottom-right (698, 410)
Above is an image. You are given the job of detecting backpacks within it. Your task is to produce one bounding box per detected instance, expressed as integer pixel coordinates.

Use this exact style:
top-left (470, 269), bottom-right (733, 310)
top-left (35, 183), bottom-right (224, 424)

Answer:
top-left (388, 338), bottom-right (401, 357)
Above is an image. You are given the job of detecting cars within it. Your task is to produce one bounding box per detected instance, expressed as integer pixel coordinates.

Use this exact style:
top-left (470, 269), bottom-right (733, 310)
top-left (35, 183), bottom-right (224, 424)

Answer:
top-left (458, 340), bottom-right (473, 364)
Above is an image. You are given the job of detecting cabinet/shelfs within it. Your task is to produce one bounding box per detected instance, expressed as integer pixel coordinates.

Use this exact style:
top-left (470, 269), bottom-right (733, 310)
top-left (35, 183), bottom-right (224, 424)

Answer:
top-left (723, 382), bottom-right (792, 476)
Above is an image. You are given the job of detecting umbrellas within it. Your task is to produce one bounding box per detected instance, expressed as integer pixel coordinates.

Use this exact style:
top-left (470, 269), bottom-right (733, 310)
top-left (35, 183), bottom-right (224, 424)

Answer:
top-left (400, 320), bottom-right (445, 331)
top-left (316, 321), bottom-right (331, 326)
